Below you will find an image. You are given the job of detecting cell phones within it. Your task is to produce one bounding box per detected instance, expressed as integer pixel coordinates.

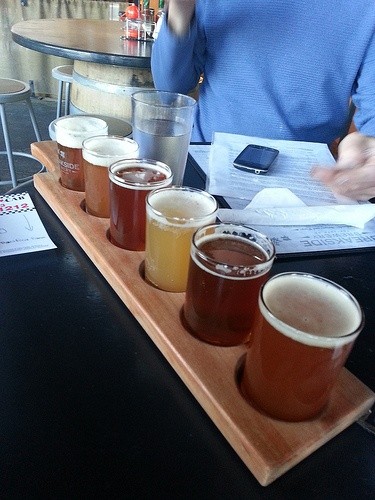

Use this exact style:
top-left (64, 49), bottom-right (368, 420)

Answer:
top-left (234, 145), bottom-right (280, 176)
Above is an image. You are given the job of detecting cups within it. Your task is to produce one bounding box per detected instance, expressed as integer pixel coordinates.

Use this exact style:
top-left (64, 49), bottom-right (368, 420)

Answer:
top-left (142, 185), bottom-right (219, 292)
top-left (182, 223), bottom-right (277, 346)
top-left (108, 157), bottom-right (175, 252)
top-left (241, 269), bottom-right (364, 423)
top-left (81, 134), bottom-right (140, 219)
top-left (52, 113), bottom-right (109, 193)
top-left (131, 89), bottom-right (197, 187)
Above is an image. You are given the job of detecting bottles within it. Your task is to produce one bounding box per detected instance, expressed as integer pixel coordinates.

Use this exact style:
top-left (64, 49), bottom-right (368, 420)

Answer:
top-left (124, 5), bottom-right (157, 41)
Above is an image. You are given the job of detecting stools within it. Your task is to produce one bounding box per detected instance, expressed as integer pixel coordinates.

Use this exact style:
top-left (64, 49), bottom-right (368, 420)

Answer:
top-left (48, 114), bottom-right (134, 142)
top-left (0, 77), bottom-right (45, 189)
top-left (52, 64), bottom-right (74, 119)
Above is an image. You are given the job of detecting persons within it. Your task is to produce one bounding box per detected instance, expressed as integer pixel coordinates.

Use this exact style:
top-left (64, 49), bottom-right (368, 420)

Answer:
top-left (149, 0), bottom-right (374, 201)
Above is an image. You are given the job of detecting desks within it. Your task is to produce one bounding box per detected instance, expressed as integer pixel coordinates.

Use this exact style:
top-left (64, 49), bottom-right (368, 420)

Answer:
top-left (10, 18), bottom-right (198, 125)
top-left (0, 143), bottom-right (375, 499)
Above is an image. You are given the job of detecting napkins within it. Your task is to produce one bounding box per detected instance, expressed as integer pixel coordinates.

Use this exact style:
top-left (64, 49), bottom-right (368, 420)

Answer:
top-left (216, 187), bottom-right (375, 229)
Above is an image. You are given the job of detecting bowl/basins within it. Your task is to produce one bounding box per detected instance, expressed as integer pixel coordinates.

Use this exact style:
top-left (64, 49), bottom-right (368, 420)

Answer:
top-left (48, 111), bottom-right (134, 140)
